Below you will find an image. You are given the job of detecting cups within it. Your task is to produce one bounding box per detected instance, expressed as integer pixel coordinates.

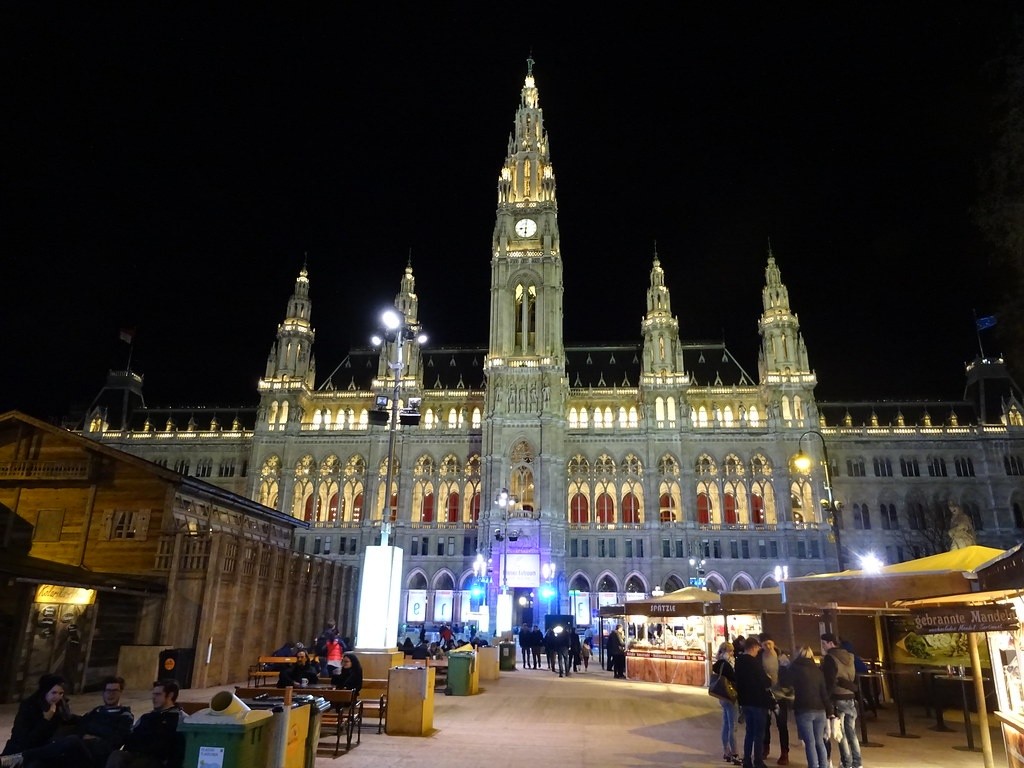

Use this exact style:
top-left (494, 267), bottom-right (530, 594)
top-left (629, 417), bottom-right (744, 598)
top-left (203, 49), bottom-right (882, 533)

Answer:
top-left (302, 678), bottom-right (309, 687)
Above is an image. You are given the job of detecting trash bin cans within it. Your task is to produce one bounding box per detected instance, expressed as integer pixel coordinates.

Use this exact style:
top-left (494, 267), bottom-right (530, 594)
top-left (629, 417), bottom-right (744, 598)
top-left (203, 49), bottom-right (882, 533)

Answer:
top-left (162, 648), bottom-right (195, 689)
top-left (178, 689), bottom-right (332, 768)
top-left (387, 665), bottom-right (434, 736)
top-left (499, 642), bottom-right (517, 671)
top-left (480, 646), bottom-right (500, 680)
top-left (444, 650), bottom-right (480, 695)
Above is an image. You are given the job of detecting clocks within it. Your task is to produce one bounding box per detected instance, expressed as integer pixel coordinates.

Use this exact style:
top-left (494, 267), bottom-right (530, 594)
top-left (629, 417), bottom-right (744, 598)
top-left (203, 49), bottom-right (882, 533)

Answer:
top-left (515, 219), bottom-right (537, 237)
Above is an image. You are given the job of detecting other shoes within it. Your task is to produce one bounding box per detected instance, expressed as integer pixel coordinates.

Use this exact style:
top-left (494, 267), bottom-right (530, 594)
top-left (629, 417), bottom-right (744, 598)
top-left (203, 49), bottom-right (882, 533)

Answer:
top-left (838, 762), bottom-right (863, 768)
top-left (742, 757), bottom-right (753, 768)
top-left (754, 759), bottom-right (768, 768)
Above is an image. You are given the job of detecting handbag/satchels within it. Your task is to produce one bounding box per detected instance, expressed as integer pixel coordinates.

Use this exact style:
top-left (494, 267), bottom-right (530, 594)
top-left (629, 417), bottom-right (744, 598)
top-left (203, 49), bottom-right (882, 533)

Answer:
top-left (708, 662), bottom-right (738, 706)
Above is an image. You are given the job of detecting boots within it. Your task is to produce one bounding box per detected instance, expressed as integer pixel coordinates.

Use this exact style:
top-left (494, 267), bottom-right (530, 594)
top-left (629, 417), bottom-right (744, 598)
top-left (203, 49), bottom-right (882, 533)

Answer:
top-left (762, 744), bottom-right (769, 760)
top-left (777, 749), bottom-right (788, 765)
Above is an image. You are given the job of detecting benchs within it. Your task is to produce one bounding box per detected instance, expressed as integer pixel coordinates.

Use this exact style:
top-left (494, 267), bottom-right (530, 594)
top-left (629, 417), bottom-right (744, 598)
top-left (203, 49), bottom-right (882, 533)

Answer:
top-left (405, 655), bottom-right (449, 682)
top-left (175, 686), bottom-right (365, 757)
top-left (313, 678), bottom-right (388, 735)
top-left (246, 656), bottom-right (323, 688)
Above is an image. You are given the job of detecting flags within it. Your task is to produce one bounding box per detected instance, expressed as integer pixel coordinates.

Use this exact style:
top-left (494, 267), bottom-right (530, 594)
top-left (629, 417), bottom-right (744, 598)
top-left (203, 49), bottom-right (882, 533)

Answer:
top-left (118, 326), bottom-right (135, 345)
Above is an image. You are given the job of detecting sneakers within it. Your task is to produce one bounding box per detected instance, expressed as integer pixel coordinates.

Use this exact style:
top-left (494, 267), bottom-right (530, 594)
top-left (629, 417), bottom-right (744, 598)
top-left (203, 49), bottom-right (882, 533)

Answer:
top-left (731, 755), bottom-right (743, 765)
top-left (723, 753), bottom-right (732, 762)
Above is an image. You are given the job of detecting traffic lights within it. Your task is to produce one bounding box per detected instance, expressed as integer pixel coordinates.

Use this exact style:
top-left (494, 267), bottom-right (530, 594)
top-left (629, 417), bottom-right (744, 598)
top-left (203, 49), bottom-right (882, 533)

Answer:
top-left (542, 588), bottom-right (553, 599)
top-left (472, 587), bottom-right (482, 599)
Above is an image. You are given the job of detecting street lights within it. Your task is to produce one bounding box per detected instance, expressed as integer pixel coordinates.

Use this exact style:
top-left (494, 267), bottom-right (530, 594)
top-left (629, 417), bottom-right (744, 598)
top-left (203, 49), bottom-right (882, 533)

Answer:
top-left (473, 552), bottom-right (488, 605)
top-left (686, 535), bottom-right (706, 589)
top-left (542, 561), bottom-right (556, 615)
top-left (793, 430), bottom-right (845, 573)
top-left (370, 305), bottom-right (430, 547)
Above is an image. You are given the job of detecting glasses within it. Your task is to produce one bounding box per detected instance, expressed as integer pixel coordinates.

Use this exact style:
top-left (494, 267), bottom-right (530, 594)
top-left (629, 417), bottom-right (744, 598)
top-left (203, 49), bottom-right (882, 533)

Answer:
top-left (342, 660), bottom-right (351, 664)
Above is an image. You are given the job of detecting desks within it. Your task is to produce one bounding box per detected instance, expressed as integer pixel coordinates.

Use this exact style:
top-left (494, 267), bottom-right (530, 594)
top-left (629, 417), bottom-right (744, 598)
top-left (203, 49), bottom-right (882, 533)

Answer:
top-left (854, 672), bottom-right (885, 748)
top-left (627, 656), bottom-right (705, 686)
top-left (874, 671), bottom-right (920, 739)
top-left (917, 670), bottom-right (959, 733)
top-left (934, 675), bottom-right (990, 751)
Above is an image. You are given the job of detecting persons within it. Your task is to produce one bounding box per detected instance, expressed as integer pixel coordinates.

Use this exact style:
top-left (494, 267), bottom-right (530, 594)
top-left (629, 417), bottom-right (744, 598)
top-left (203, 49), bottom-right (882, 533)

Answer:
top-left (710, 631), bottom-right (874, 768)
top-left (331, 654), bottom-right (363, 703)
top-left (438, 622), bottom-right (447, 644)
top-left (606, 623), bottom-right (626, 679)
top-left (567, 626), bottom-right (594, 672)
top-left (469, 625), bottom-right (477, 641)
top-left (519, 622), bottom-right (571, 679)
top-left (397, 636), bottom-right (467, 661)
top-left (262, 618), bottom-right (351, 678)
top-left (276, 650), bottom-right (318, 689)
top-left (454, 625), bottom-right (460, 633)
top-left (416, 625), bottom-right (426, 646)
top-left (104, 677), bottom-right (186, 768)
top-left (441, 626), bottom-right (455, 647)
top-left (946, 494), bottom-right (977, 551)
top-left (0, 674), bottom-right (75, 756)
top-left (1, 675), bottom-right (135, 768)
top-left (473, 637), bottom-right (488, 647)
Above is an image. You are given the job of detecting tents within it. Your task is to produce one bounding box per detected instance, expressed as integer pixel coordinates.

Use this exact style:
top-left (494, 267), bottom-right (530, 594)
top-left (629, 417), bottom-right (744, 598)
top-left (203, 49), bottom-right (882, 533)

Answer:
top-left (712, 578), bottom-right (904, 659)
top-left (595, 604), bottom-right (626, 670)
top-left (624, 587), bottom-right (759, 689)
top-left (779, 546), bottom-right (1023, 768)
